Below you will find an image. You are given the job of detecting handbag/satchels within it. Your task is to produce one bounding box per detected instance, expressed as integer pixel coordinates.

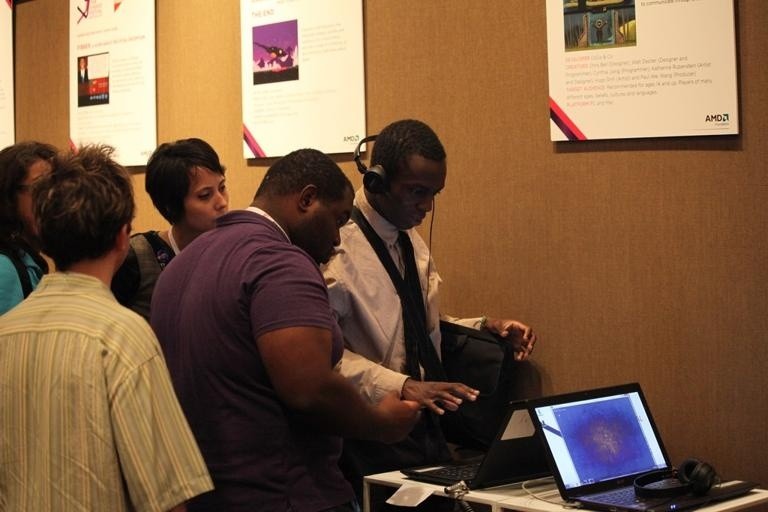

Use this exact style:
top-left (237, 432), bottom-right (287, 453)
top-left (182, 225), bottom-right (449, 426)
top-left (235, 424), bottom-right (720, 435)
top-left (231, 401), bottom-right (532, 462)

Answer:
top-left (406, 321), bottom-right (516, 449)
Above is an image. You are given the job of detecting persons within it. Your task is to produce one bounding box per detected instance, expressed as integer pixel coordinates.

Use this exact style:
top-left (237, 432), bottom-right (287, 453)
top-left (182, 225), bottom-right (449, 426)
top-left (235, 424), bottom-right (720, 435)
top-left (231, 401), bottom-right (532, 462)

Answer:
top-left (1, 120), bottom-right (537, 511)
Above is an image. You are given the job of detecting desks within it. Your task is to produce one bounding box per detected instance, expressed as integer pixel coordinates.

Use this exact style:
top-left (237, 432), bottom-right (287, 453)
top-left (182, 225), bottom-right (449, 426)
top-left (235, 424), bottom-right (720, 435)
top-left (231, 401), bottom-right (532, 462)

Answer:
top-left (360, 465), bottom-right (768, 512)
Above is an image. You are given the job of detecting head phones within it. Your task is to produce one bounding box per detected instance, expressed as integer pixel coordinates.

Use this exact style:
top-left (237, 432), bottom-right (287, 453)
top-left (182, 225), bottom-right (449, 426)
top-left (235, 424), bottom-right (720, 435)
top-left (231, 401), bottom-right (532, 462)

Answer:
top-left (353, 136), bottom-right (390, 196)
top-left (633, 457), bottom-right (716, 498)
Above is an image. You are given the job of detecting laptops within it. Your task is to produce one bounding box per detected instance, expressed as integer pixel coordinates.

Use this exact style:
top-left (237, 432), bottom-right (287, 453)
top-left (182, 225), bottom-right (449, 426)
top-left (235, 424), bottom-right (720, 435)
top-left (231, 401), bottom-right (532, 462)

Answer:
top-left (525, 382), bottom-right (761, 512)
top-left (399, 397), bottom-right (553, 490)
